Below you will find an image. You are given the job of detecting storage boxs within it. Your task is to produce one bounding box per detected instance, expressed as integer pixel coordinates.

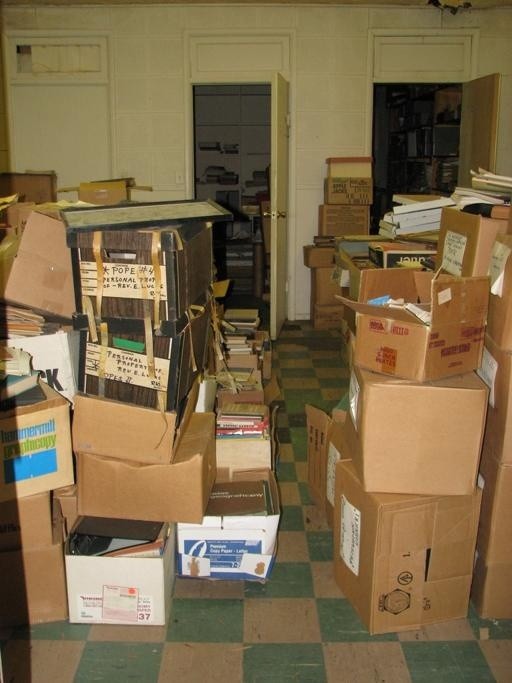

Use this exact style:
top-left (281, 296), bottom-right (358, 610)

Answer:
top-left (304, 156), bottom-right (510, 637)
top-left (1, 171), bottom-right (281, 630)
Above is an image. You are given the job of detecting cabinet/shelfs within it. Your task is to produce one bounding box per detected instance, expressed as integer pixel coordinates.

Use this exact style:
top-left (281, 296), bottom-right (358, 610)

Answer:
top-left (385, 84), bottom-right (462, 210)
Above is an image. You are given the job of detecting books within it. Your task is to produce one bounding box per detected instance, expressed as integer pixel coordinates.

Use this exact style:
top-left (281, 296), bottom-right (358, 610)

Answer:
top-left (217, 319), bottom-right (273, 439)
top-left (377, 165), bottom-right (511, 240)
top-left (204, 480), bottom-right (268, 516)
top-left (0, 303), bottom-right (45, 407)
